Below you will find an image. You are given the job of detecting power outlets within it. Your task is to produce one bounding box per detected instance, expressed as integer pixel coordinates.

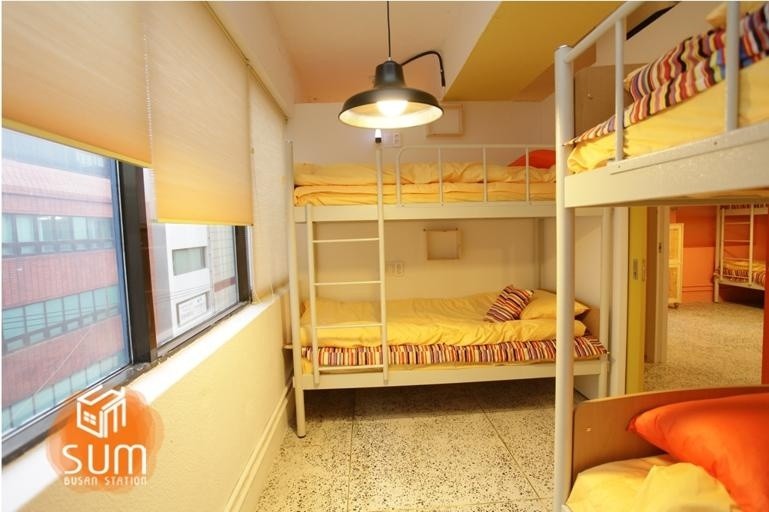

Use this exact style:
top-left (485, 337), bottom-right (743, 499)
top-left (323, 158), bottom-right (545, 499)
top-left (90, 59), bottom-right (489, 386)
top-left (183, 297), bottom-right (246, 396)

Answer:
top-left (393, 133), bottom-right (400, 145)
top-left (395, 263), bottom-right (404, 276)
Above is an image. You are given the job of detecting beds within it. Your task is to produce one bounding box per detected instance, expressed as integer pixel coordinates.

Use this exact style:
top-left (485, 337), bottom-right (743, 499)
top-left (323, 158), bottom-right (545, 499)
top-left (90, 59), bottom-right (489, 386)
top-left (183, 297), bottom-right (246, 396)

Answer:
top-left (551, 1), bottom-right (769, 512)
top-left (286, 139), bottom-right (613, 439)
top-left (713, 203), bottom-right (769, 303)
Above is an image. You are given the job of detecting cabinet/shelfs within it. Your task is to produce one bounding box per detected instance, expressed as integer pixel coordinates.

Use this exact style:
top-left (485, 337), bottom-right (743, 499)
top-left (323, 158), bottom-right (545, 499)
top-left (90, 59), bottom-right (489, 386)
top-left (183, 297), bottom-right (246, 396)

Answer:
top-left (667, 222), bottom-right (684, 309)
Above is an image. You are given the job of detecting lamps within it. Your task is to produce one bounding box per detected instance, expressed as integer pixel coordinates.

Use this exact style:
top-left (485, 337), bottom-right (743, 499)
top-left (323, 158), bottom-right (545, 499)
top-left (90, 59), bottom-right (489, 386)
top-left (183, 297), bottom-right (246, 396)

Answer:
top-left (338, 1), bottom-right (446, 130)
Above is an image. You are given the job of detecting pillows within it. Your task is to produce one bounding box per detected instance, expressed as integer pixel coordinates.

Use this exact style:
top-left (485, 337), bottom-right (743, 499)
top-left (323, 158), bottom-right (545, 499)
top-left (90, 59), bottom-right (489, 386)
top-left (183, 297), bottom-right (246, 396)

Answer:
top-left (520, 289), bottom-right (591, 319)
top-left (508, 150), bottom-right (556, 169)
top-left (490, 288), bottom-right (534, 322)
top-left (706, 1), bottom-right (760, 26)
top-left (627, 393), bottom-right (769, 512)
top-left (485, 284), bottom-right (515, 321)
